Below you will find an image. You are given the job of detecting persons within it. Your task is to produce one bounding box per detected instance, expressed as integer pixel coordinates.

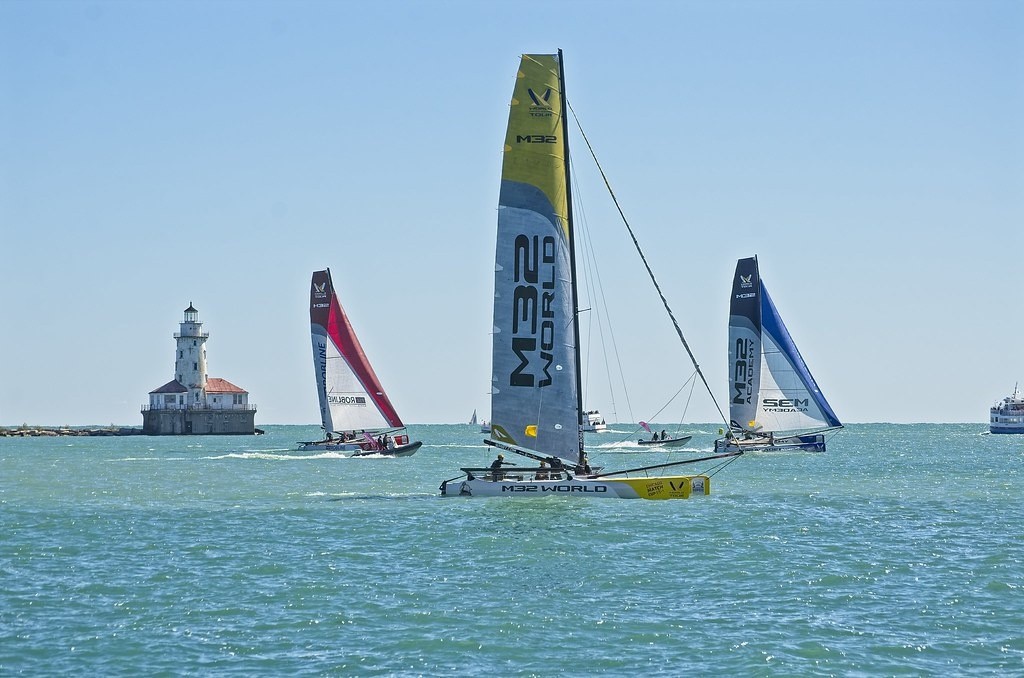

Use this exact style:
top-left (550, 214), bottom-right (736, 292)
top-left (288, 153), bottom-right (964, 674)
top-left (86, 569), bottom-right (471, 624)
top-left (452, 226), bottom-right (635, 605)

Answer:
top-left (535, 461), bottom-right (549, 479)
top-left (378, 434), bottom-right (390, 450)
top-left (652, 431), bottom-right (658, 440)
top-left (491, 454), bottom-right (517, 468)
top-left (661, 430), bottom-right (667, 440)
top-left (575, 459), bottom-right (592, 476)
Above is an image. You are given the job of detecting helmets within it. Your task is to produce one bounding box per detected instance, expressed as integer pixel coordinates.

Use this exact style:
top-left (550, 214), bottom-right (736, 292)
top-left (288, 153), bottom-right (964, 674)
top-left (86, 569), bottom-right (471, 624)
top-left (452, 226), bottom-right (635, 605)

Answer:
top-left (540, 462), bottom-right (546, 464)
top-left (584, 458), bottom-right (588, 462)
top-left (498, 454), bottom-right (504, 460)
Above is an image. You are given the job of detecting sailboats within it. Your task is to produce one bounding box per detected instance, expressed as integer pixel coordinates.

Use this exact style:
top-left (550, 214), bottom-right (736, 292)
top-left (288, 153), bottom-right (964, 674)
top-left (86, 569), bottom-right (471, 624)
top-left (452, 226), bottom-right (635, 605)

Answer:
top-left (295, 266), bottom-right (423, 459)
top-left (440, 47), bottom-right (713, 501)
top-left (710, 254), bottom-right (849, 456)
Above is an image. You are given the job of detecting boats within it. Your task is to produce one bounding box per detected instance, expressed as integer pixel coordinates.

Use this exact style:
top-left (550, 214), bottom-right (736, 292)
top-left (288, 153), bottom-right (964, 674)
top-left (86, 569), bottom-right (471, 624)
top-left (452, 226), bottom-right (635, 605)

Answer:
top-left (989, 382), bottom-right (1024, 433)
top-left (581, 409), bottom-right (606, 434)
top-left (638, 434), bottom-right (692, 448)
